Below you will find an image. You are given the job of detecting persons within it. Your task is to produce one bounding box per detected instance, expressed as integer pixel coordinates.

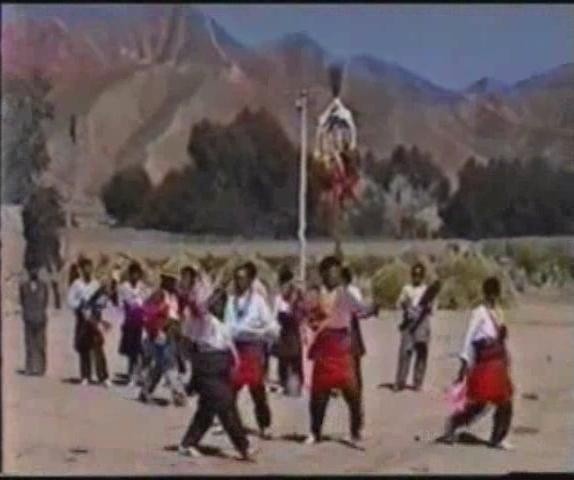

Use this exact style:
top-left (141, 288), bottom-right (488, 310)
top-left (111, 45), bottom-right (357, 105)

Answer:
top-left (442, 278), bottom-right (515, 452)
top-left (395, 264), bottom-right (439, 390)
top-left (19, 265), bottom-right (48, 377)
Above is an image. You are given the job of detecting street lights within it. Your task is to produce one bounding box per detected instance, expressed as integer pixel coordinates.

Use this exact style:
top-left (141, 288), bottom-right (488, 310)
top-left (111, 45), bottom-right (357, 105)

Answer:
top-left (293, 87), bottom-right (312, 281)
